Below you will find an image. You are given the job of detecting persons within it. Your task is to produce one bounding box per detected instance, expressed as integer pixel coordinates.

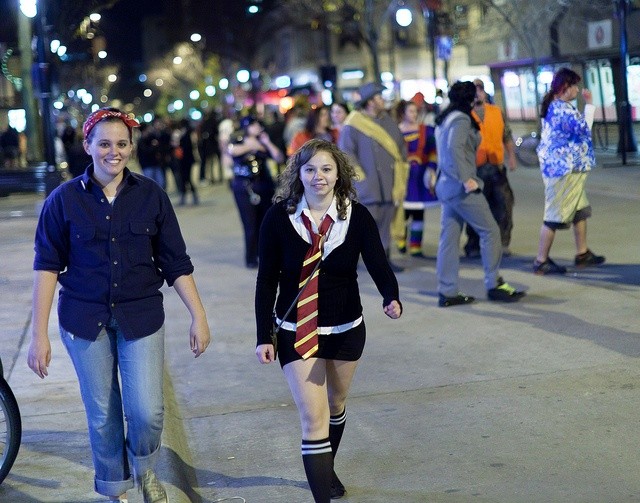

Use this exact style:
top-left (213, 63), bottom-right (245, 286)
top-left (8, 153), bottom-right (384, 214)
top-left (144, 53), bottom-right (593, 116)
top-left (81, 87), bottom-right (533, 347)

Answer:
top-left (27, 107), bottom-right (210, 503)
top-left (227, 116), bottom-right (284, 267)
top-left (137, 107), bottom-right (334, 206)
top-left (340, 83), bottom-right (409, 273)
top-left (291, 106), bottom-right (336, 157)
top-left (462, 78), bottom-right (518, 259)
top-left (435, 81), bottom-right (526, 307)
top-left (331, 104), bottom-right (351, 140)
top-left (255, 139), bottom-right (403, 502)
top-left (534, 69), bottom-right (606, 274)
top-left (397, 99), bottom-right (438, 256)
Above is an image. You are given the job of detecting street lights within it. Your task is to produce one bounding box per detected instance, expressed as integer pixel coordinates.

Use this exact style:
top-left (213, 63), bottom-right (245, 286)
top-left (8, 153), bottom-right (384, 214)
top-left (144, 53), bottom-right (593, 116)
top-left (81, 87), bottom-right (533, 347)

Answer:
top-left (18, 0), bottom-right (61, 199)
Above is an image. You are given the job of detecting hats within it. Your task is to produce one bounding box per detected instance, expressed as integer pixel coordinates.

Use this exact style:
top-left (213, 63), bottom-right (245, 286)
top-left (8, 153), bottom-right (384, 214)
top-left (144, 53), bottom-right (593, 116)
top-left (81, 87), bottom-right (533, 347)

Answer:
top-left (360, 83), bottom-right (387, 100)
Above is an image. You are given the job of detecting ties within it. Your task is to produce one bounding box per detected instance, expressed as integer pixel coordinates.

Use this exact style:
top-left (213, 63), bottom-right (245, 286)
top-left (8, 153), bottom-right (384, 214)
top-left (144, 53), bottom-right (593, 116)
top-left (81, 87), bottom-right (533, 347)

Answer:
top-left (294, 211), bottom-right (333, 360)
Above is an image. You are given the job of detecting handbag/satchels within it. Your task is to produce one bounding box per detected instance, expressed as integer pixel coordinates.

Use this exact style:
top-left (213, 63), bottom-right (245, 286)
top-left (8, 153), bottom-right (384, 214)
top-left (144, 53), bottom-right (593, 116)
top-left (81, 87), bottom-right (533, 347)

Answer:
top-left (270, 221), bottom-right (335, 360)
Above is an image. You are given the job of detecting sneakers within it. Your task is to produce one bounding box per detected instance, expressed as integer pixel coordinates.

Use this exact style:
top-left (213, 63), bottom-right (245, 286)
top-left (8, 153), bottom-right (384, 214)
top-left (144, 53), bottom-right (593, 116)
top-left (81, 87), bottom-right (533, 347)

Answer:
top-left (534, 258), bottom-right (567, 274)
top-left (468, 250), bottom-right (480, 258)
top-left (488, 278), bottom-right (524, 301)
top-left (576, 250), bottom-right (605, 265)
top-left (439, 292), bottom-right (474, 307)
top-left (503, 246), bottom-right (513, 255)
top-left (110, 499), bottom-right (125, 503)
top-left (138, 470), bottom-right (168, 503)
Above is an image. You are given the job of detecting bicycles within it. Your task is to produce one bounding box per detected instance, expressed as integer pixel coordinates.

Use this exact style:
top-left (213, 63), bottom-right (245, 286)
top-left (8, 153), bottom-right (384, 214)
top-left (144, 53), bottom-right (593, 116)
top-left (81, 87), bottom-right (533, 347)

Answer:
top-left (515, 134), bottom-right (542, 167)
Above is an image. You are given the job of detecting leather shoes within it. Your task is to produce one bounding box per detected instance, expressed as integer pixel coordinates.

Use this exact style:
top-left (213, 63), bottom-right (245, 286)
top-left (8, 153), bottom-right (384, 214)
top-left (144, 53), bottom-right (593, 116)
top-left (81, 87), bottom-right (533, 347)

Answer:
top-left (331, 471), bottom-right (345, 499)
